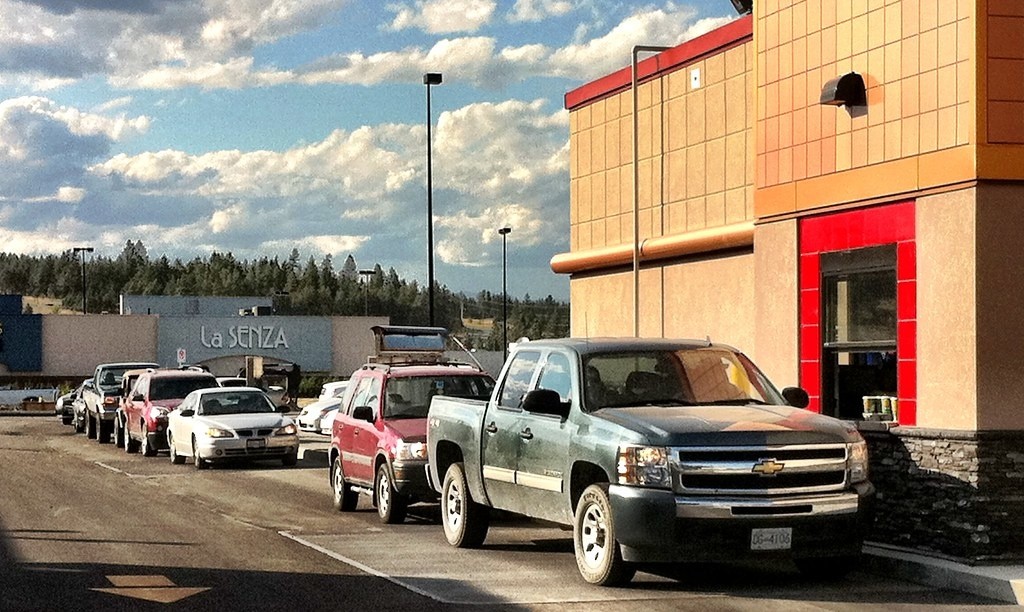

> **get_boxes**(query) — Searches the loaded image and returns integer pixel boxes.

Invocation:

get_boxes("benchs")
[21,401,55,411]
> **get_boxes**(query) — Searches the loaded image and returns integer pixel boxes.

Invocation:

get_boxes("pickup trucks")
[425,336,872,589]
[83,362,161,444]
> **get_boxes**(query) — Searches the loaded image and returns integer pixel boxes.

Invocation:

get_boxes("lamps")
[820,71,869,107]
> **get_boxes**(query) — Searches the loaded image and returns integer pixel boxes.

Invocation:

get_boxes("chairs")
[389,393,408,416]
[204,399,222,411]
[238,399,253,412]
[628,371,664,400]
[105,372,116,382]
[426,389,465,411]
[583,366,606,407]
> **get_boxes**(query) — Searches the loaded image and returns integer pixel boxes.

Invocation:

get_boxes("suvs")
[122,366,222,459]
[328,351,498,527]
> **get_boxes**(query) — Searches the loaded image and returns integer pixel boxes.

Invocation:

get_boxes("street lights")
[73,247,94,314]
[498,226,512,361]
[360,270,377,317]
[423,72,443,324]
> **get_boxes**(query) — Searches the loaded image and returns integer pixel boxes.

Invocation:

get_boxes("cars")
[216,376,248,388]
[263,381,350,436]
[54,377,94,434]
[166,387,300,470]
